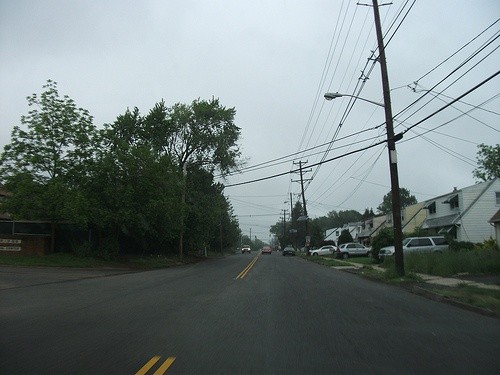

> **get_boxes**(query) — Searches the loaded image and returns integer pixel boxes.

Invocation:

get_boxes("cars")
[241,244,251,254]
[261,245,273,255]
[282,247,296,256]
[309,245,338,257]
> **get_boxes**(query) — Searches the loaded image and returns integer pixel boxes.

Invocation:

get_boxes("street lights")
[178,159,223,261]
[323,91,404,277]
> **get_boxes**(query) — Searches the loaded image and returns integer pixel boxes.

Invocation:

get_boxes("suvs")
[336,243,373,259]
[377,236,450,264]
